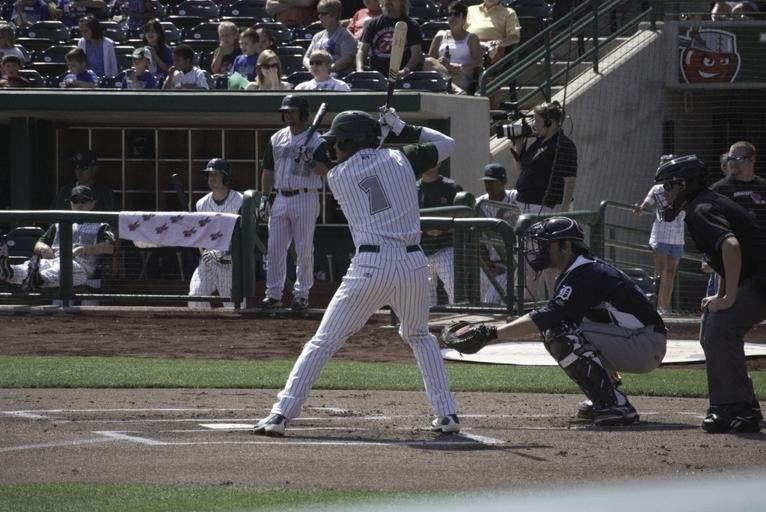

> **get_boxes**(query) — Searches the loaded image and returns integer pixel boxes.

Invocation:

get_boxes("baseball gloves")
[440,320,496,354]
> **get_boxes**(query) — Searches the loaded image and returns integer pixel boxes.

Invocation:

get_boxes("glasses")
[447,12,461,17]
[71,198,89,203]
[663,182,679,191]
[310,60,326,65]
[317,12,329,16]
[261,63,278,69]
[724,155,750,163]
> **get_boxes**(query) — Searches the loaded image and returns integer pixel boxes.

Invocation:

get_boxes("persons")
[257,95,331,307]
[414,102,577,305]
[447,216,668,427]
[711,0,761,21]
[630,139,766,433]
[186,158,246,308]
[0,0,518,95]
[49,152,115,308]
[252,111,461,442]
[0,185,115,289]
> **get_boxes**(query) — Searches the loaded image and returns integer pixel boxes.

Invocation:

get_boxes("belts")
[277,188,307,196]
[221,259,231,264]
[360,245,421,252]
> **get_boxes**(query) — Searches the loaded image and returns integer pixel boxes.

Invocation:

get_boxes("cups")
[111,76,121,87]
[99,76,111,87]
[216,77,228,89]
[46,78,57,87]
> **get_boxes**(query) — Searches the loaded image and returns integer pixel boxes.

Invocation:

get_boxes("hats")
[126,48,152,64]
[64,185,94,202]
[480,164,506,181]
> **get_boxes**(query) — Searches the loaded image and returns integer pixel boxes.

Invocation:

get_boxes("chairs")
[0,226,46,297]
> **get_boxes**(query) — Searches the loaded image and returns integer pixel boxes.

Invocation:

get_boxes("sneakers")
[262,298,283,307]
[702,408,759,433]
[21,254,40,293]
[595,405,638,424]
[291,296,310,310]
[578,401,605,419]
[0,246,13,283]
[253,413,287,436]
[431,414,460,432]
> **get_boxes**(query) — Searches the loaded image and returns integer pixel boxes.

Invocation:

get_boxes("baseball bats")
[296,103,329,162]
[386,21,408,110]
[171,172,204,257]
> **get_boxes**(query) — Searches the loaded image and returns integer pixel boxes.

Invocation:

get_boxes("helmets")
[525,216,584,281]
[320,110,381,150]
[201,158,232,185]
[279,95,308,123]
[653,154,707,222]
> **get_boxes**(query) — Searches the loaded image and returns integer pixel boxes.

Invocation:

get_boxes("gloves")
[259,196,271,222]
[296,144,314,165]
[379,105,398,128]
[202,249,223,265]
